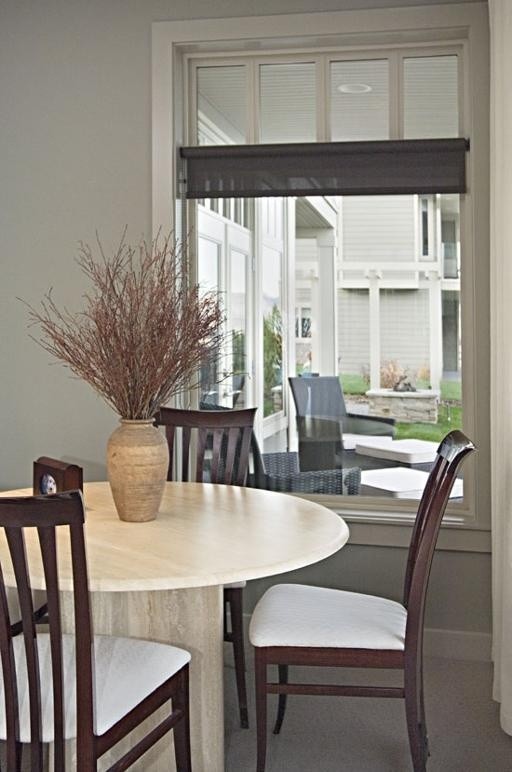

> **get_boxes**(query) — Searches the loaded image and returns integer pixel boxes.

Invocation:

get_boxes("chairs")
[202,395,362,495]
[156,402,261,731]
[245,425,481,772]
[0,482,190,769]
[285,374,398,470]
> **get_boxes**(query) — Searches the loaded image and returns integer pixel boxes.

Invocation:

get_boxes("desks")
[351,436,445,472]
[0,464,350,771]
[347,462,465,501]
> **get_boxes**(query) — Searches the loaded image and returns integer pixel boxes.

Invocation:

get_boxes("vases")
[104,415,172,525]
